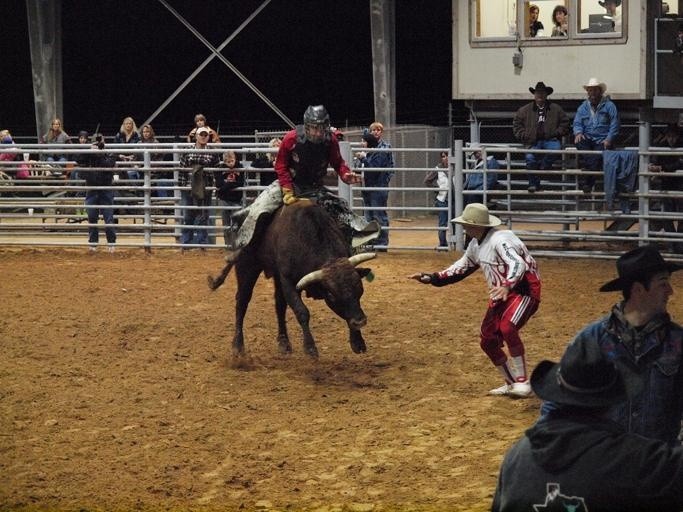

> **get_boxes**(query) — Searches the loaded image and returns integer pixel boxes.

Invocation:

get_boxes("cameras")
[94,136,104,149]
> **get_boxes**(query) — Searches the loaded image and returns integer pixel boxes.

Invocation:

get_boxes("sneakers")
[488,384,511,395]
[528,186,537,193]
[224,248,249,263]
[582,185,593,193]
[508,380,535,397]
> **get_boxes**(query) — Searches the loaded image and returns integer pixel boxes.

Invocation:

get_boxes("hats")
[450,203,502,227]
[467,142,486,157]
[599,245,683,292]
[529,82,553,95]
[91,134,105,144]
[361,134,379,147]
[662,122,682,134]
[530,333,628,407]
[196,127,210,135]
[583,78,607,94]
[599,0,621,7]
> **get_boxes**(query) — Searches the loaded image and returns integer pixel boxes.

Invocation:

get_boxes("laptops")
[589,13,614,34]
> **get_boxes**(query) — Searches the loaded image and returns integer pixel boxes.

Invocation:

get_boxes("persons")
[353,132,389,253]
[370,122,396,181]
[600,1,622,31]
[463,143,499,203]
[129,126,171,215]
[250,138,282,194]
[553,6,567,37]
[0,131,19,160]
[406,203,541,398]
[178,128,220,250]
[529,4,544,37]
[217,151,246,249]
[43,119,71,167]
[113,117,139,160]
[83,135,116,252]
[78,131,88,144]
[649,123,683,255]
[491,338,683,512]
[541,247,682,443]
[514,82,569,191]
[424,151,455,247]
[186,114,220,143]
[573,78,619,198]
[224,106,381,263]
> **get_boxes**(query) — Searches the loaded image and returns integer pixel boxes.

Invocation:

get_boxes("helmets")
[303,105,330,144]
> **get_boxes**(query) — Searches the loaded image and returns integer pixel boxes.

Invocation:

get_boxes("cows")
[205,194,379,365]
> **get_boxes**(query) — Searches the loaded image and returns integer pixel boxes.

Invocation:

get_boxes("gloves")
[282,187,298,205]
